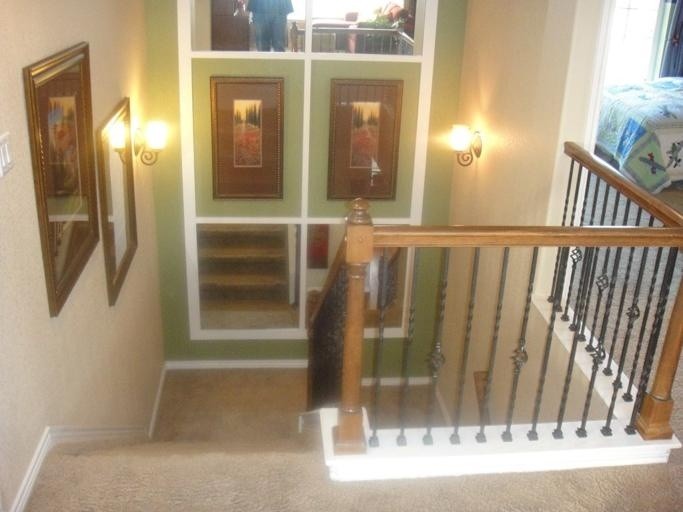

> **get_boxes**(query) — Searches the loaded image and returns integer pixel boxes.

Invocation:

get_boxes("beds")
[595,75,683,196]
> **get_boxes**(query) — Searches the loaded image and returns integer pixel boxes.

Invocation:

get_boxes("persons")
[245,0,294,51]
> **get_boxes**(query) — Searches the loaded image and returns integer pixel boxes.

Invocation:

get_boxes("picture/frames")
[96,97,139,306]
[23,41,103,318]
[329,79,404,201]
[209,76,285,200]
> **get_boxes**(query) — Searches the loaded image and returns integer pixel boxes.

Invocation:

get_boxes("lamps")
[134,123,170,166]
[450,122,482,167]
